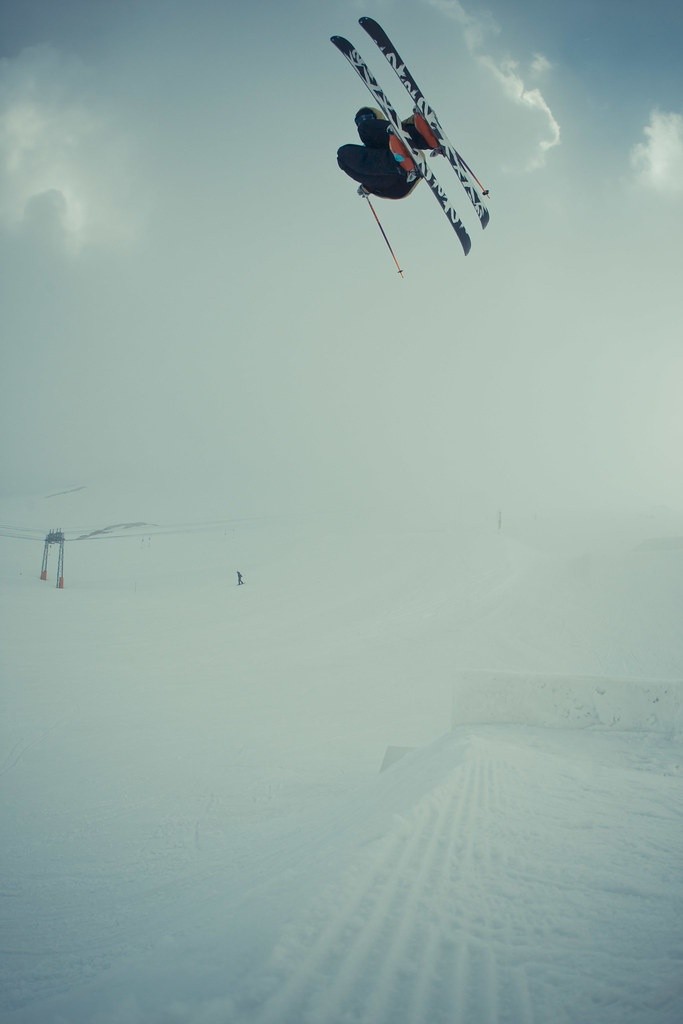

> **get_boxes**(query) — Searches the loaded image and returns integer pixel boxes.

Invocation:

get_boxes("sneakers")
[413,107,441,158]
[387,126,417,182]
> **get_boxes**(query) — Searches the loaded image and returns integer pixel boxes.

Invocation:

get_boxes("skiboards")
[330,17,489,256]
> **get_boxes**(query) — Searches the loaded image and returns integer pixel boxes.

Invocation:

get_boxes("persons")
[337,106,440,199]
[236,571,243,586]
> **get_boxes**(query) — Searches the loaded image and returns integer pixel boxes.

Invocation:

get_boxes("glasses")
[354,114,373,124]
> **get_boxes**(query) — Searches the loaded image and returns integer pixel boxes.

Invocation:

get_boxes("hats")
[356,107,385,120]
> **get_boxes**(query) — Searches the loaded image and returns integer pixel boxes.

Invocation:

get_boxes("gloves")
[357,185,370,198]
